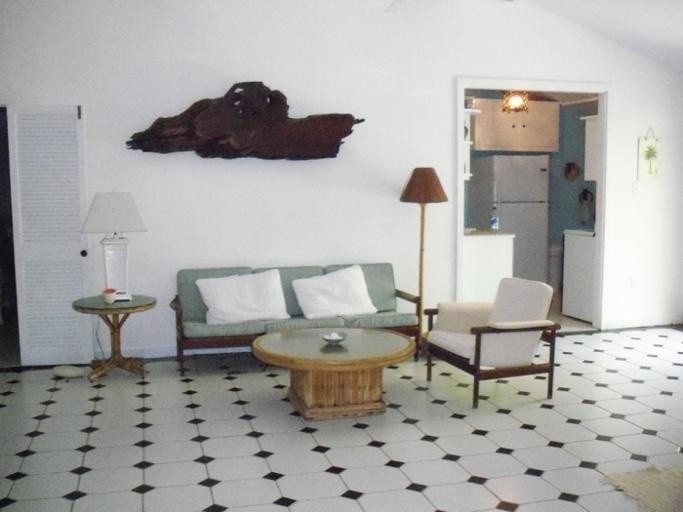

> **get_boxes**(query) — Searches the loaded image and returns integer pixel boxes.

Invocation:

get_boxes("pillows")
[195,263,379,324]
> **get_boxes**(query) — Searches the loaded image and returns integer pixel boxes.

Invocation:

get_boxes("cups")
[103,288,116,304]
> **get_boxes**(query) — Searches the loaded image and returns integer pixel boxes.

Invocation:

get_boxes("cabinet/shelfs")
[466,97,559,153]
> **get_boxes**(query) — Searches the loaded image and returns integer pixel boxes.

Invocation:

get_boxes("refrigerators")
[467,154,550,284]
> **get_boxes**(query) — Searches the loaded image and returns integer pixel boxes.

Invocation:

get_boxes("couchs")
[168,262,422,376]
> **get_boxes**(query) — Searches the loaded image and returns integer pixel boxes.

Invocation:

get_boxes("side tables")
[72,295,157,382]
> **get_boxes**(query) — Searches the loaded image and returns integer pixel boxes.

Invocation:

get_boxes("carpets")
[605,466,683,512]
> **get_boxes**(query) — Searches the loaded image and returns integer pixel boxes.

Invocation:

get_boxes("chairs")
[423,277,561,409]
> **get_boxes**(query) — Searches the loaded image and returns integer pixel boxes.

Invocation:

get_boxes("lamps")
[399,168,450,361]
[81,190,148,301]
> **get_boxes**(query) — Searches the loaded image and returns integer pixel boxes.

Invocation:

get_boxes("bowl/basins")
[319,330,348,345]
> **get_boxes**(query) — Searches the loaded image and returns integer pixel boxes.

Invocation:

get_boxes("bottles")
[488,206,500,234]
